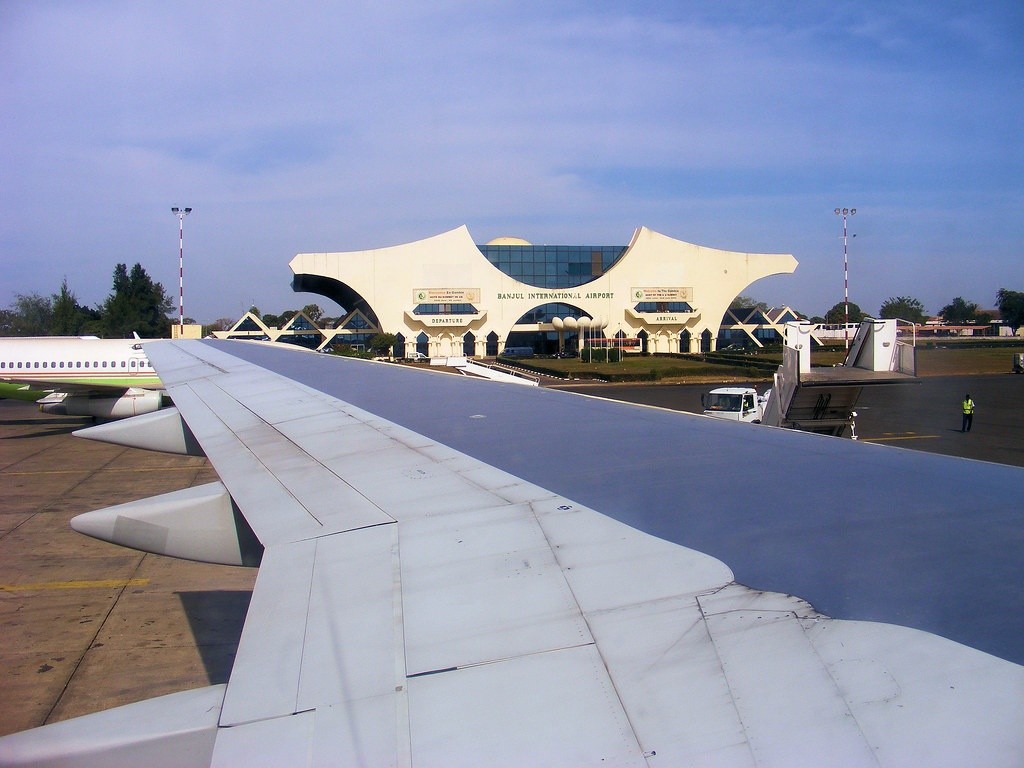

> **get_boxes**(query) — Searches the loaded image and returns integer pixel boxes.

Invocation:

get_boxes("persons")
[961,394,974,432]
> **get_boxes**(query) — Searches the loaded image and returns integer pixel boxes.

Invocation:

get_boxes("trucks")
[701,386,772,425]
[1012,353,1024,373]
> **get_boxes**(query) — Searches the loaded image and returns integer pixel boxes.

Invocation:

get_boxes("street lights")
[170,206,192,340]
[833,208,858,361]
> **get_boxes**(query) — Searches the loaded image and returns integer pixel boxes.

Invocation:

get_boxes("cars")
[721,342,751,350]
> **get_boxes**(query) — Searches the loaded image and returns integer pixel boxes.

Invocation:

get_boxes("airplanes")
[1,329,319,418]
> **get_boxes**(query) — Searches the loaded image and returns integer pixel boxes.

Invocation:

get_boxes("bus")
[574,337,642,355]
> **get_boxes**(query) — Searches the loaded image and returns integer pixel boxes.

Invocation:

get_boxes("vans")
[498,346,534,357]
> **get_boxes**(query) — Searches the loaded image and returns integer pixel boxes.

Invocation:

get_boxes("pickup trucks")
[405,353,431,361]
